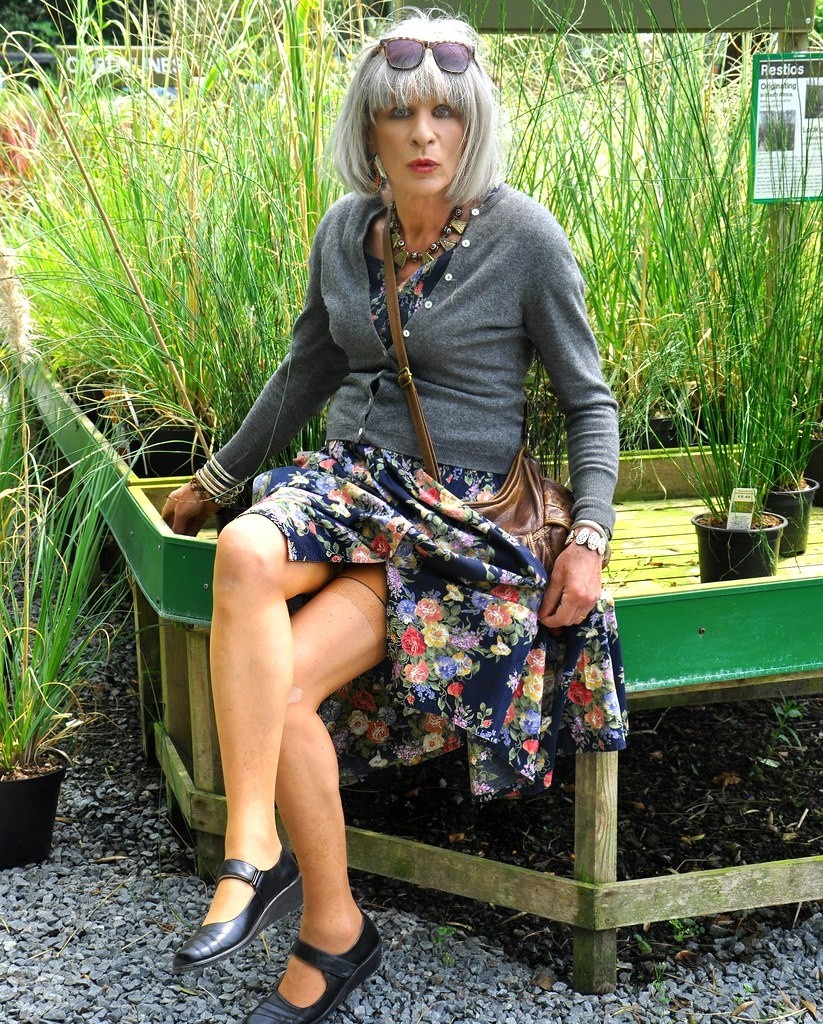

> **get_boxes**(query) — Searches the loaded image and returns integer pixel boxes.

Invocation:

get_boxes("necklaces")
[389,199,466,269]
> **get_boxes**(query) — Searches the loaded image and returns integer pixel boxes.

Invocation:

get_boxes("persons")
[162,11,630,1024]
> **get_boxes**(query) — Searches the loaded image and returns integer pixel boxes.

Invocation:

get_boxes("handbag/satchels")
[464,451,612,579]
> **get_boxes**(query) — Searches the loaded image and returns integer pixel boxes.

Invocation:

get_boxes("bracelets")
[189,455,246,507]
[563,527,612,571]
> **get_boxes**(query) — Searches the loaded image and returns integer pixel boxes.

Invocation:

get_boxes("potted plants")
[0,388,151,872]
[591,0,823,584]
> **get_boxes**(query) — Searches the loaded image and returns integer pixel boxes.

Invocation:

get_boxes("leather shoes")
[243,909,382,1024]
[172,846,308,970]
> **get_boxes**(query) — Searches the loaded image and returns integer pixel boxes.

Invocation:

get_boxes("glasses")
[371,37,475,74]
